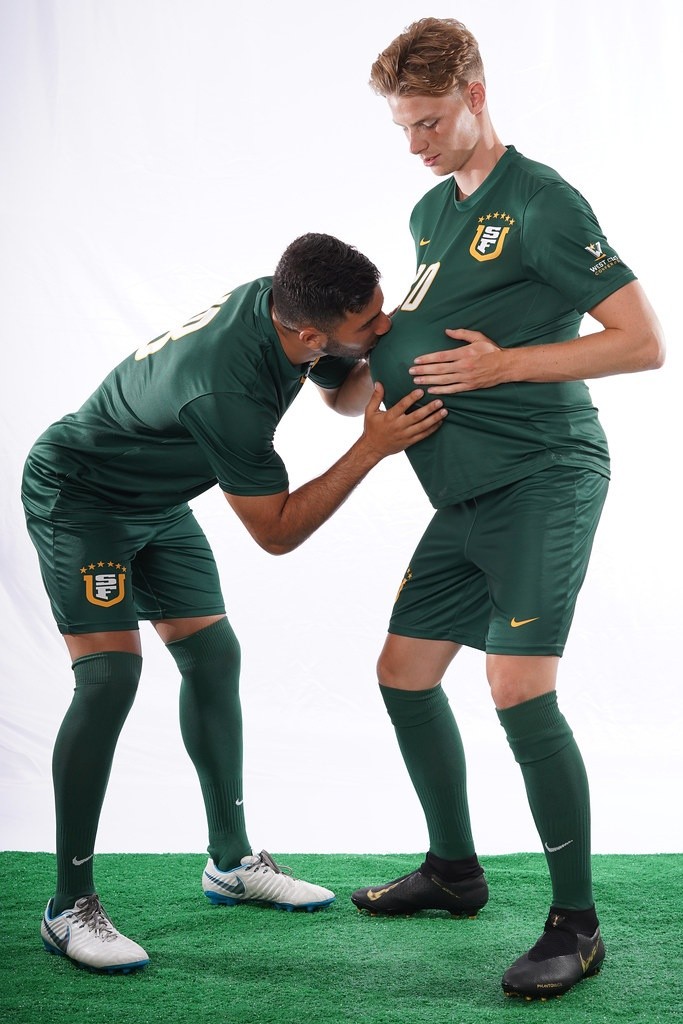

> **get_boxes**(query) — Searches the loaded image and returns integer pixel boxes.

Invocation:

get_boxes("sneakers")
[500,913,604,1001]
[202,849,335,913]
[350,854,489,919]
[41,892,149,974]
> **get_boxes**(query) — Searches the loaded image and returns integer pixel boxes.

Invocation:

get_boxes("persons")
[21,234,446,975]
[370,19,663,1003]
